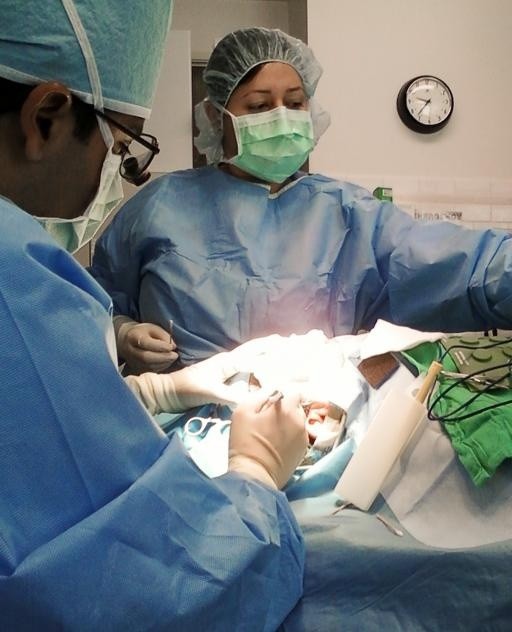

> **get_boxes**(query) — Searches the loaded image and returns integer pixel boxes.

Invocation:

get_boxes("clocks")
[397,74,453,134]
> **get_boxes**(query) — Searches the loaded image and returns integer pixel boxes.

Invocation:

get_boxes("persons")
[0,1,312,630]
[83,23,511,380]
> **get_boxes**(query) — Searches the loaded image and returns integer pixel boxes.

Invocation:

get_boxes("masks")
[212,102,313,183]
[30,0,124,254]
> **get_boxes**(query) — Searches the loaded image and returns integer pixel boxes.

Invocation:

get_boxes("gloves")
[149,353,263,412]
[117,317,179,374]
[229,387,308,490]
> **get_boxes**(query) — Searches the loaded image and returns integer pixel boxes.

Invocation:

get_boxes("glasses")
[73,93,159,186]
